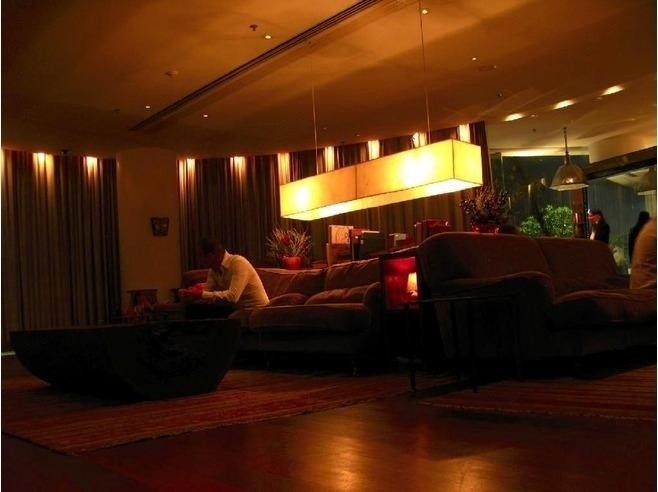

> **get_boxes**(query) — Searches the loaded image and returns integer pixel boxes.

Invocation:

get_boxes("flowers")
[264,223,314,267]
[457,182,512,223]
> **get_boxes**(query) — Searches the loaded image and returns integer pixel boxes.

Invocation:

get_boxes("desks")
[8,317,243,407]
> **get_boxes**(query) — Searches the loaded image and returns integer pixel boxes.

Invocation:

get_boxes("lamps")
[150,218,168,236]
[548,126,589,193]
[278,0,483,222]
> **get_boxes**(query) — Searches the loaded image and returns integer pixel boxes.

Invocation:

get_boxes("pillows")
[180,268,210,289]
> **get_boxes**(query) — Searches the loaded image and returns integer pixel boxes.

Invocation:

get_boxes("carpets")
[2,367,460,456]
[415,362,656,425]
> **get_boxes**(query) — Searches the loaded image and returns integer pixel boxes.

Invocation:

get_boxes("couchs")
[407,233,656,393]
[233,257,381,377]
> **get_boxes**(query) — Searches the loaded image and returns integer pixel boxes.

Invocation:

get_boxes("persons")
[178,239,270,337]
[628,212,649,263]
[588,209,609,244]
[629,217,657,290]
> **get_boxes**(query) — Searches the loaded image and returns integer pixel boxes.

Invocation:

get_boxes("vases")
[471,218,503,233]
[278,256,302,270]
